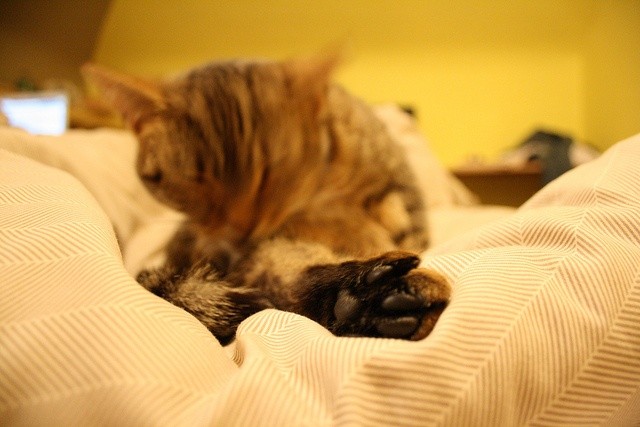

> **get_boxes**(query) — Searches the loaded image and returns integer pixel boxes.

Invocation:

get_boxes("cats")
[69,53,453,341]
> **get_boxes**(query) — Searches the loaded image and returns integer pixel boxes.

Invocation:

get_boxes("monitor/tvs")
[0,87,72,138]
